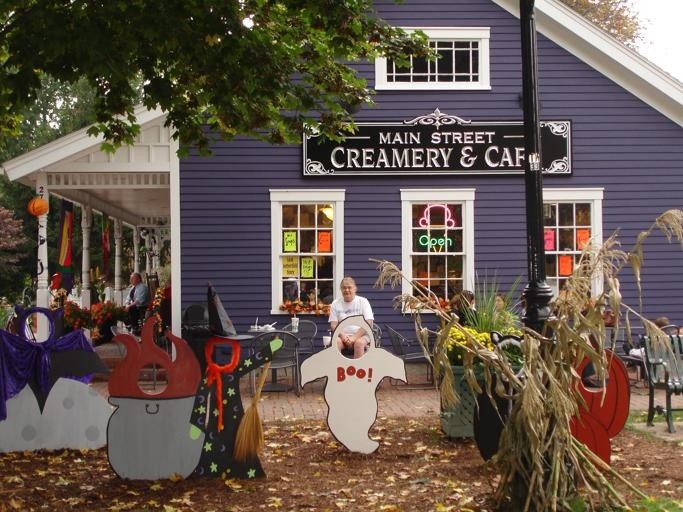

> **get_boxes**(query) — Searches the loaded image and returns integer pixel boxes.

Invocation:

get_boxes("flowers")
[62,286,169,345]
[436,323,523,367]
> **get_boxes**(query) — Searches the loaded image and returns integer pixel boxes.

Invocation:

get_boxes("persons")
[488,293,509,329]
[123,272,148,336]
[622,316,670,360]
[458,290,479,328]
[444,294,464,327]
[327,277,374,359]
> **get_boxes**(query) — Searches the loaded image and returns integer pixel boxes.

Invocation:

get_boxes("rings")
[347,340,349,342]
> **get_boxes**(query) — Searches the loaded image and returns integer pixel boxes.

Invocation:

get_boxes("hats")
[192,284,255,343]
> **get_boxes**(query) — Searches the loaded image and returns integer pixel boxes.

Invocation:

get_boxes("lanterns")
[26,197,48,216]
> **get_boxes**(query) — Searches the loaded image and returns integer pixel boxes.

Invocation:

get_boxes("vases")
[438,364,491,444]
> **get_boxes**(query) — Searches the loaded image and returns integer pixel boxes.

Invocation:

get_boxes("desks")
[222,334,255,395]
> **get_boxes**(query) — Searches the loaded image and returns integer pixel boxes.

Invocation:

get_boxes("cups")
[290,318,299,334]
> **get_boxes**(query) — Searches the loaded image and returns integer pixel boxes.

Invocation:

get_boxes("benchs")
[640,333,683,434]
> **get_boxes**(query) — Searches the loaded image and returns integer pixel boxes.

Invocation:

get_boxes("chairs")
[107,325,170,391]
[384,322,435,391]
[615,323,678,383]
[248,318,318,397]
